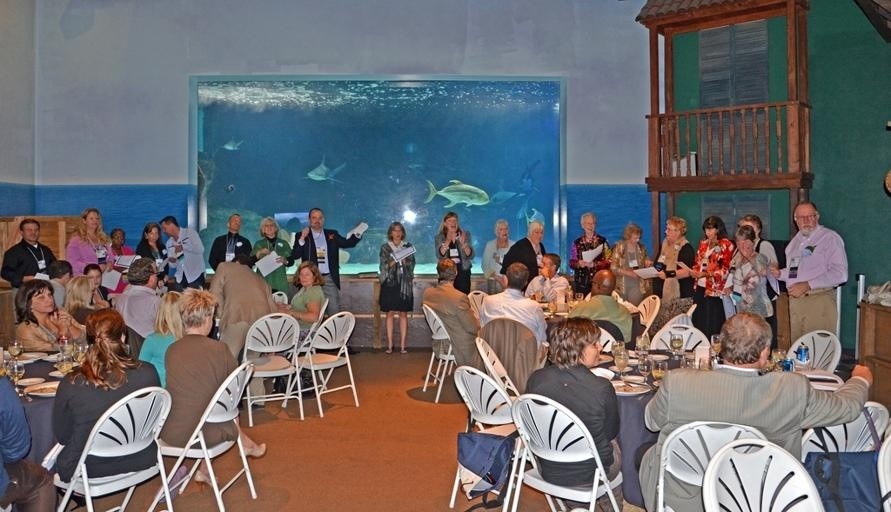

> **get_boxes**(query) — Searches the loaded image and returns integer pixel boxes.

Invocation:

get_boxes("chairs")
[701,437,827,512]
[448,366,526,511]
[464,289,728,351]
[422,302,455,403]
[271,292,333,390]
[510,390,624,511]
[473,315,544,400]
[786,325,891,512]
[239,311,305,428]
[285,311,360,418]
[145,358,258,511]
[54,385,175,511]
[655,420,769,511]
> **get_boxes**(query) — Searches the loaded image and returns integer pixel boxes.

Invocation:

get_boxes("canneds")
[782,358,794,372]
[796,346,809,362]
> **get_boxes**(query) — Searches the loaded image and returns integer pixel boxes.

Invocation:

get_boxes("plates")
[13,350,78,398]
[589,350,669,397]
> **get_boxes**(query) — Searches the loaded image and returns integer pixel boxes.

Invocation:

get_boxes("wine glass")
[610,334,786,386]
[0,337,26,390]
[56,335,94,375]
[534,287,584,315]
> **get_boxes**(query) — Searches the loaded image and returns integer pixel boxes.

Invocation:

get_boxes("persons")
[379,222,415,354]
[1,208,364,512]
[524,316,624,512]
[434,202,847,359]
[632,312,873,512]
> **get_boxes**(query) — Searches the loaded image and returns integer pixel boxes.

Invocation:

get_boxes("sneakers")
[347,346,361,355]
[251,403,265,412]
[400,345,408,355]
[385,345,395,354]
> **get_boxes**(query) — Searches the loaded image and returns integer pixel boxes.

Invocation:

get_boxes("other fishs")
[224,183,237,194]
[491,190,526,206]
[302,153,347,187]
[422,179,490,209]
[520,160,542,194]
[523,208,545,228]
[224,136,245,153]
[517,198,528,220]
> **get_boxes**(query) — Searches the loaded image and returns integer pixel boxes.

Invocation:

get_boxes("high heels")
[239,442,266,459]
[194,470,219,492]
